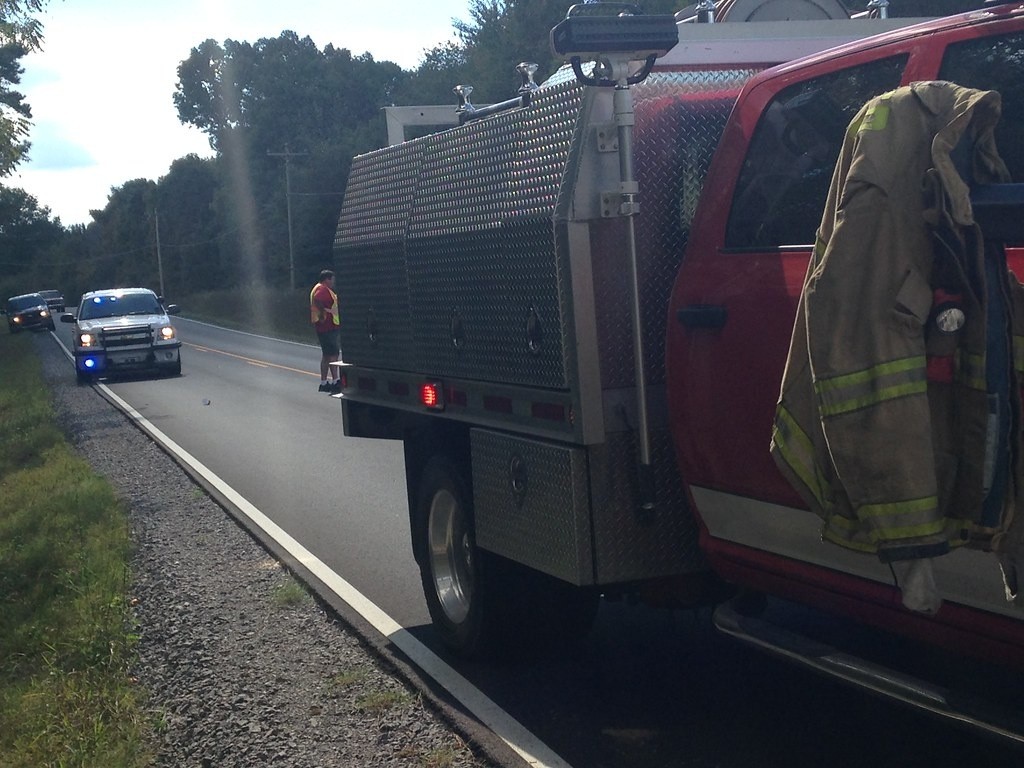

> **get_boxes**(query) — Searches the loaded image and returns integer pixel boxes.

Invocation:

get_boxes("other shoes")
[318,381,333,392]
[330,380,344,396]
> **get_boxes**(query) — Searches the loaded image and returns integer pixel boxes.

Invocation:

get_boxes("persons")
[309,270,341,393]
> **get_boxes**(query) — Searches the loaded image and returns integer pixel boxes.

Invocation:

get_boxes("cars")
[38,289,67,313]
[60,287,183,381]
[2,293,56,334]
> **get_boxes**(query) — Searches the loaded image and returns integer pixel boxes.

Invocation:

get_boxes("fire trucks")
[317,1,1024,768]
